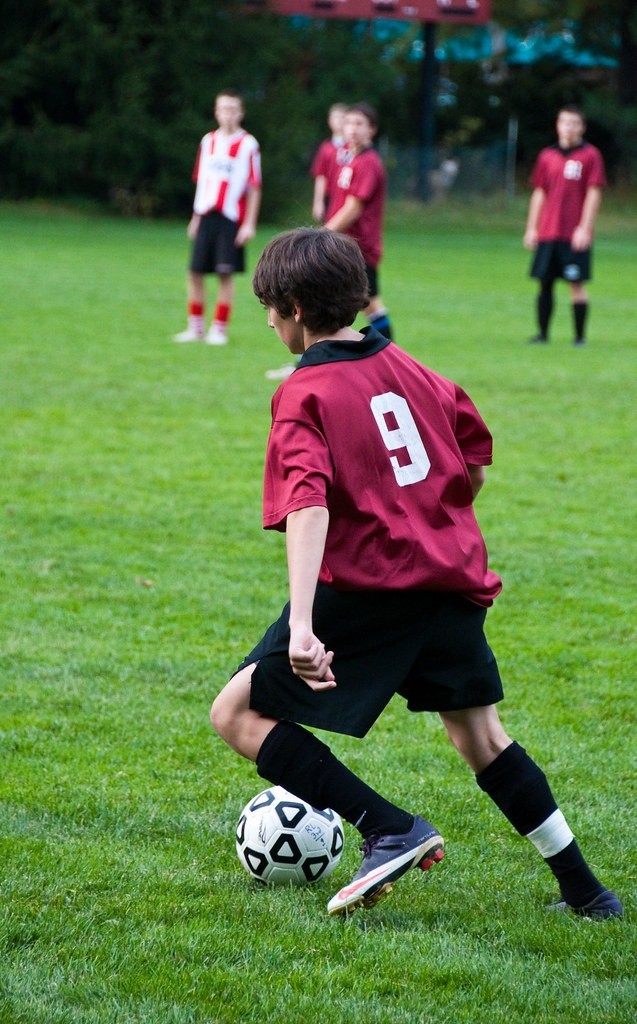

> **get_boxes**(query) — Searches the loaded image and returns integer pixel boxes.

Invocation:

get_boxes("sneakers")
[543,889,624,920]
[323,812,448,921]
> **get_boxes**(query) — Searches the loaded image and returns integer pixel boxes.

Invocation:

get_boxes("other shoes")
[265,364,299,383]
[206,328,229,347]
[171,326,201,344]
[573,338,588,347]
[530,332,550,343]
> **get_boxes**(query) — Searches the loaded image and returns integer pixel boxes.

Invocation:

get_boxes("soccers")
[234,783,345,886]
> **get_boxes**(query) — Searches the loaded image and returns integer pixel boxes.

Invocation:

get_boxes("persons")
[309,100,602,347]
[178,91,262,343]
[211,226,625,925]
